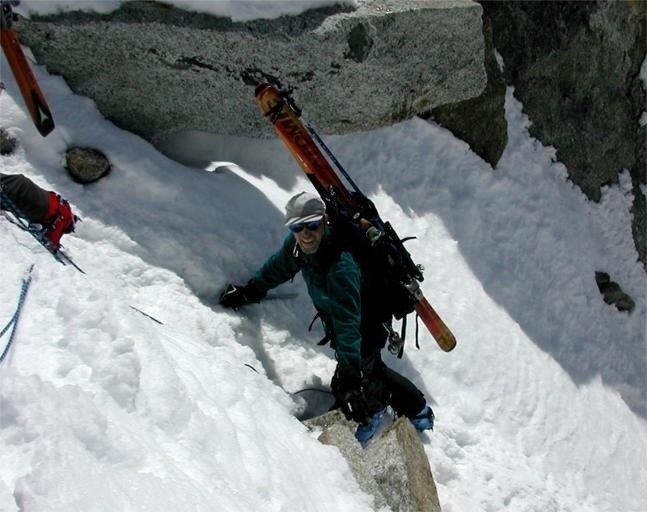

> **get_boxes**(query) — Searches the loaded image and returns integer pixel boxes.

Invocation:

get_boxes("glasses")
[289,222,320,234]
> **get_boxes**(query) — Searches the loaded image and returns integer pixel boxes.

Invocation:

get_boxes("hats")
[284,193,327,227]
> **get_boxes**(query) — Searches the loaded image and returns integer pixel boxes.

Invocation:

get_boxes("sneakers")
[412,404,435,430]
[355,407,389,444]
[41,194,74,254]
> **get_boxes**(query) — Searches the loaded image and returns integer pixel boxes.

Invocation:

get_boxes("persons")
[219,192,433,448]
[0,174,78,254]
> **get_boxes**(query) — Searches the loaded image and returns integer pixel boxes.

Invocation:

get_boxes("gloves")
[336,380,372,426]
[220,278,264,310]
